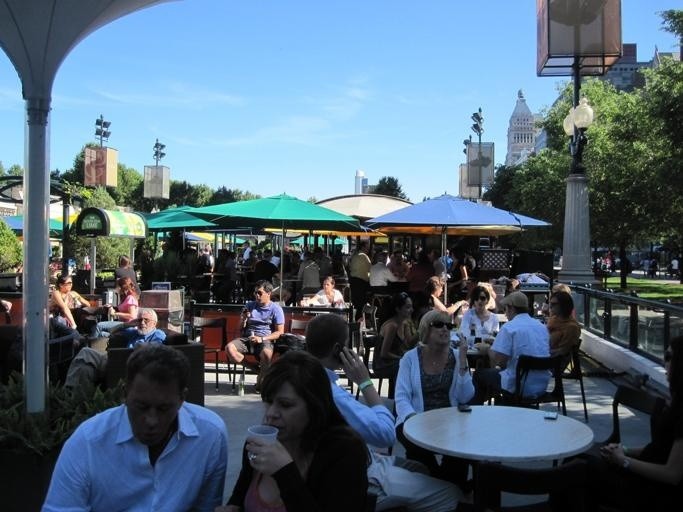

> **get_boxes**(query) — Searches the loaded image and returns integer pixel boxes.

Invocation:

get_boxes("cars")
[627,255,640,270]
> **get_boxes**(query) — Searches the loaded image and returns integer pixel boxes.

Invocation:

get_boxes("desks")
[402,405,594,512]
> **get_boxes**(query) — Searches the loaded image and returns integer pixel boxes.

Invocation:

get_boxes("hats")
[499,291,529,308]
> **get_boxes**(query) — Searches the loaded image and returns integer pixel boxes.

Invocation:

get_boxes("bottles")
[238,379,245,396]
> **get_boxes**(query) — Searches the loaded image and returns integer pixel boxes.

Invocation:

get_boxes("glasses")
[475,296,487,301]
[254,291,262,296]
[429,321,453,330]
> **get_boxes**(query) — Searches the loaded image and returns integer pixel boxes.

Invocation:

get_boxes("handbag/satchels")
[274,333,306,352]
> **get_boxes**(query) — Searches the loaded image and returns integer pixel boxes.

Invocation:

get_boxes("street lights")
[152,139,166,212]
[462,135,473,201]
[561,99,595,282]
[471,107,487,200]
[93,114,110,191]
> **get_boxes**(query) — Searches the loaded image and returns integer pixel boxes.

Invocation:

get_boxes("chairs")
[335,322,360,394]
[86,327,206,407]
[190,315,231,392]
[561,384,666,463]
[356,303,379,337]
[356,335,392,401]
[0,324,74,386]
[81,305,112,348]
[558,339,589,423]
[289,319,307,334]
[174,256,352,305]
[231,327,280,395]
[490,354,570,416]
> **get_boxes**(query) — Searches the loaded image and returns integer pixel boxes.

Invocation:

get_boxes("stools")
[370,294,394,313]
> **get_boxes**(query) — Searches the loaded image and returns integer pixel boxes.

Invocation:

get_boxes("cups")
[245,425,280,441]
[466,335,474,349]
[301,296,349,310]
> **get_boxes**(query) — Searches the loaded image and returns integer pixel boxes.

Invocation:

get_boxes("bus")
[591,249,620,272]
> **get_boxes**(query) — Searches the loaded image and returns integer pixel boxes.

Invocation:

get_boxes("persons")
[1,239,683,512]
